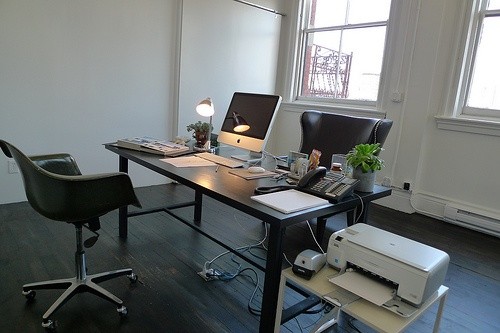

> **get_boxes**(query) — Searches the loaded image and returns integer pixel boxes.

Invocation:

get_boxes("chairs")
[298,111,393,246]
[0,138,142,330]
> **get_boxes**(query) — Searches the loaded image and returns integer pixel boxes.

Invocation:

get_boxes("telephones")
[297,166,360,203]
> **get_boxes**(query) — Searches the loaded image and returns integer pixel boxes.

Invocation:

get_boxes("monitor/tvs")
[217,92,282,162]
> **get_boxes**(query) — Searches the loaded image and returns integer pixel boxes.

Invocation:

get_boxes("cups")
[332,154,353,178]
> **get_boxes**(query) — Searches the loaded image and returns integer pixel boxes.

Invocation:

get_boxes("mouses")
[248,166,265,173]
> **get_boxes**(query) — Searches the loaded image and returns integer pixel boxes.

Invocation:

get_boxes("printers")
[326,223,449,316]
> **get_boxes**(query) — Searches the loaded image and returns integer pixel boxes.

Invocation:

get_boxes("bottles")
[330,163,342,175]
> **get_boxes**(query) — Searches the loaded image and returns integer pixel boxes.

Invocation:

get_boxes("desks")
[103,140,392,333]
[274,263,449,333]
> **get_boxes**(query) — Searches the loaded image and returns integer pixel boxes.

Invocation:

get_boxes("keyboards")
[193,152,244,169]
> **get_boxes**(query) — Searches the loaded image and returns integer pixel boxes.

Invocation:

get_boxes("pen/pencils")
[216,165,220,172]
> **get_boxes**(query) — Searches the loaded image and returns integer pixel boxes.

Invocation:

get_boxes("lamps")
[193,97,215,152]
[226,111,251,132]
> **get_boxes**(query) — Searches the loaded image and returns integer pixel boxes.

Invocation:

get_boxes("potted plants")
[186,120,214,145]
[345,143,385,194]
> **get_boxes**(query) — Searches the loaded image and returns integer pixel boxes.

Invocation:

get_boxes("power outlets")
[8,159,18,174]
[197,269,220,281]
